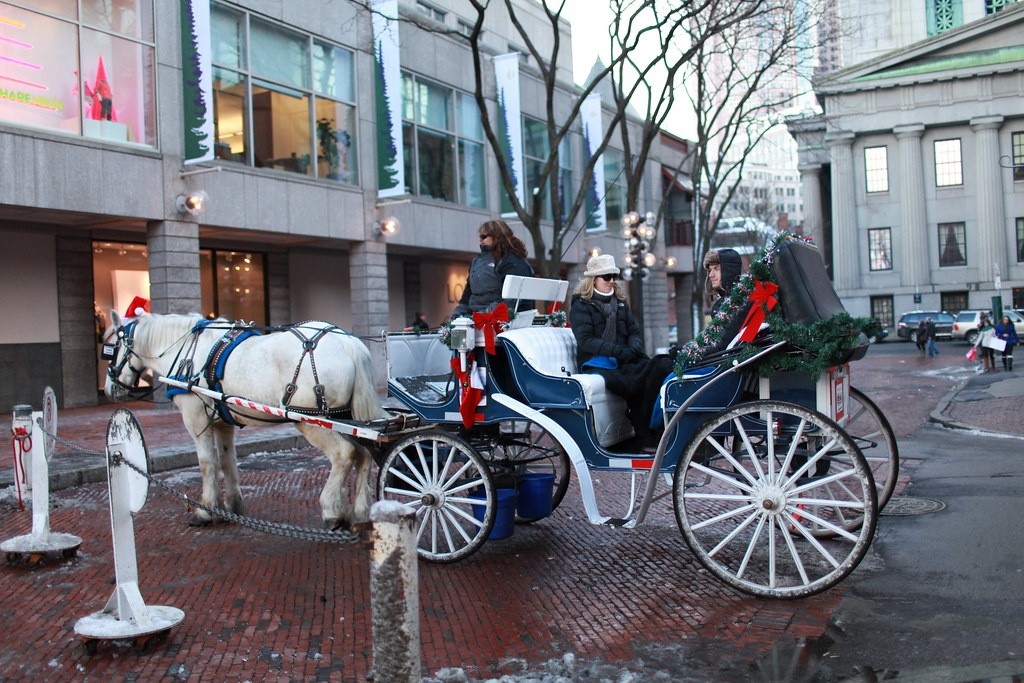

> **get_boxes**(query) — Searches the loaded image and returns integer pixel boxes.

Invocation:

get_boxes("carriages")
[101,235,899,603]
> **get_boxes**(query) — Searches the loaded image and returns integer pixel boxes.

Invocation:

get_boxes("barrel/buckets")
[510,443,557,519]
[467,465,517,541]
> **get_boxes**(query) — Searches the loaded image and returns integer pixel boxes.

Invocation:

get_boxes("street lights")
[622,210,656,354]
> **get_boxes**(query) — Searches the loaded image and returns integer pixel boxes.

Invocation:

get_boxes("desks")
[268,158,308,174]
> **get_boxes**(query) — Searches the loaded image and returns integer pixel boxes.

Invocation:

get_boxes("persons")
[569,253,658,452]
[916,317,940,360]
[995,315,1019,372]
[629,249,742,422]
[413,311,429,334]
[974,311,997,371]
[451,220,536,327]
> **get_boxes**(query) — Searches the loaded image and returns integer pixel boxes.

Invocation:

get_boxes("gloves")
[669,346,684,360]
[618,347,636,363]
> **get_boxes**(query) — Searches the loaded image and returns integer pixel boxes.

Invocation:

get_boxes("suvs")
[951,309,1024,346]
[897,311,957,343]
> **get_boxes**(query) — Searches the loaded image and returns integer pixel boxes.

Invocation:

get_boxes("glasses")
[479,234,491,240]
[596,275,617,282]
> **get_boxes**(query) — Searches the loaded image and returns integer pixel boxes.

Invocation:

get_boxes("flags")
[73,56,117,121]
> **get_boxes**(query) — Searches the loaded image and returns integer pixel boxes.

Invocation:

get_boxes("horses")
[100,308,383,545]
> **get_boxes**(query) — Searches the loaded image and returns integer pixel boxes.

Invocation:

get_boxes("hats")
[584,253,620,277]
[704,248,742,296]
[416,310,424,317]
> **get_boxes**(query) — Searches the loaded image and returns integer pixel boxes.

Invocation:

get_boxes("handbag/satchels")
[920,332,928,344]
[966,348,977,362]
[988,334,1007,351]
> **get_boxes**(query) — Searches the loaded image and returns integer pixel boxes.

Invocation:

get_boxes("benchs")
[458,274,569,347]
[650,321,772,433]
[496,327,636,448]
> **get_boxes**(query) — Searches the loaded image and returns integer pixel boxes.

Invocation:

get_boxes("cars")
[869,327,888,344]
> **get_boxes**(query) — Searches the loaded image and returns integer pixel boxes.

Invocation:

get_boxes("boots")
[1001,356,1007,371]
[1007,355,1013,371]
[990,357,996,370]
[984,358,989,372]
[626,408,659,453]
